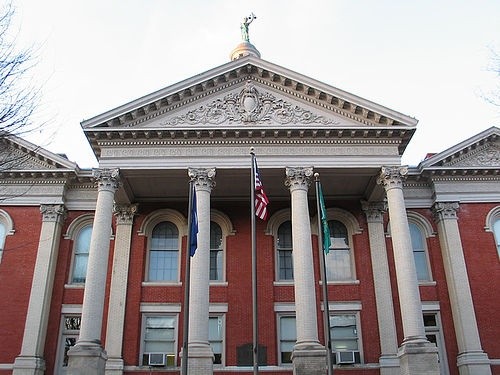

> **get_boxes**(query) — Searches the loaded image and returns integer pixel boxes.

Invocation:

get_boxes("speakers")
[149,353,165,365]
[335,352,355,365]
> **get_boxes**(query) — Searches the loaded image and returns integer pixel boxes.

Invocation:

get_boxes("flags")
[190,185,199,257]
[319,182,331,257]
[255,158,269,221]
